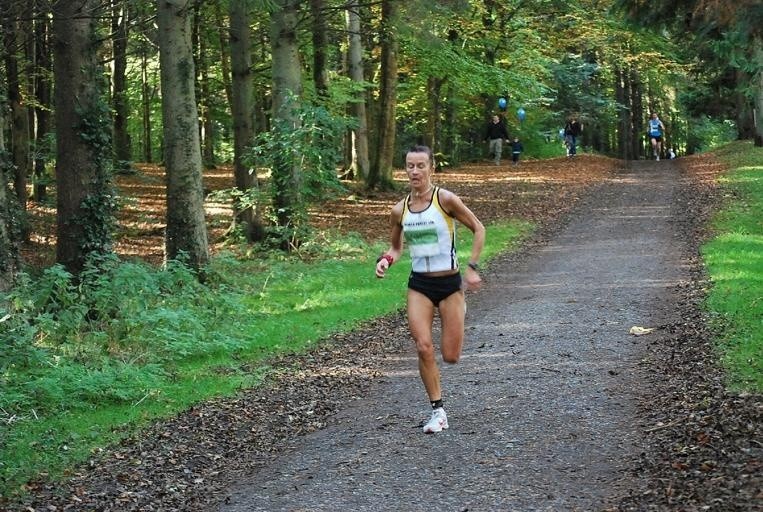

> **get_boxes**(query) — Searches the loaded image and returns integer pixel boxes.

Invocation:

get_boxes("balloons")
[498,98,507,109]
[517,108,525,120]
[559,128,565,138]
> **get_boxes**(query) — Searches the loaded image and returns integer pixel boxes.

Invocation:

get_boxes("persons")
[507,135,524,166]
[564,114,584,157]
[647,112,666,162]
[375,144,486,433]
[483,114,510,166]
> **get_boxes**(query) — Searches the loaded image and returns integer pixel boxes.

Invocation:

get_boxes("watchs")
[469,262,478,269]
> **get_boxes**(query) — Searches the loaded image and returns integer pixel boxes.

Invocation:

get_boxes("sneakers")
[423,408,449,433]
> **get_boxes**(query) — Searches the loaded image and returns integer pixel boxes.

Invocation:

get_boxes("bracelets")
[377,255,393,267]
